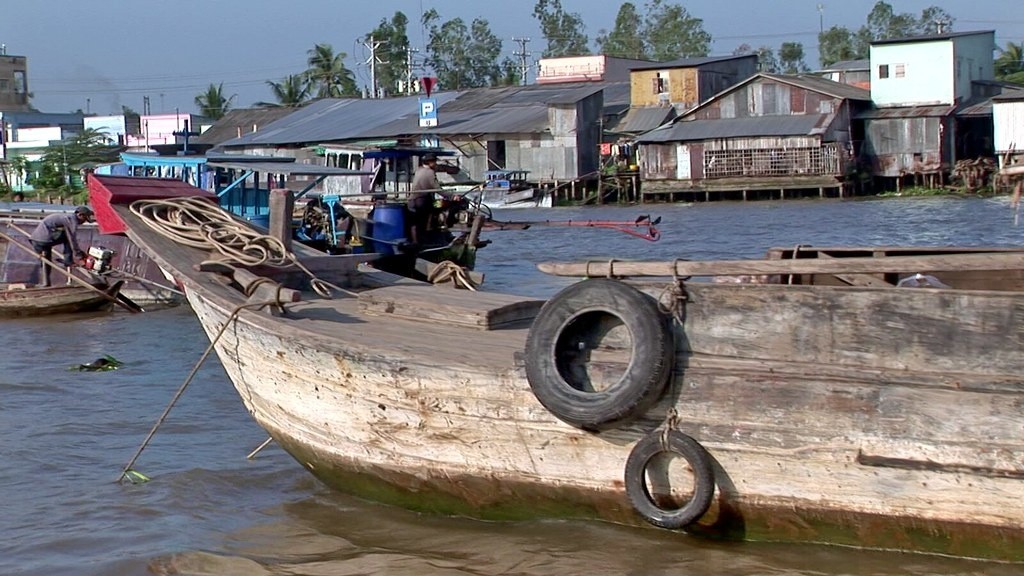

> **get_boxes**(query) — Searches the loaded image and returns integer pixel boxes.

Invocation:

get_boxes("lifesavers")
[623,428,715,529]
[522,277,669,434]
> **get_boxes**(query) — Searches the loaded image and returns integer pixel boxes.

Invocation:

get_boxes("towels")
[600,143,610,155]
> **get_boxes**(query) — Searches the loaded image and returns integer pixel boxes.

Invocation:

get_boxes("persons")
[406,153,461,246]
[12,193,23,201]
[29,207,93,286]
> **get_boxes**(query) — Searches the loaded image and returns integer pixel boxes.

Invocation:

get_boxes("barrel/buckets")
[244,214,269,231]
[371,202,406,253]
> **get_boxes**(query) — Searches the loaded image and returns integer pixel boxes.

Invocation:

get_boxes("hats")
[77,206,93,223]
[423,153,441,160]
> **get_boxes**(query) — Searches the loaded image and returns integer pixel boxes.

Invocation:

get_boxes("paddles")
[0,219,146,316]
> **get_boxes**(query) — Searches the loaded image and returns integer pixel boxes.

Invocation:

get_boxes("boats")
[94,140,663,277]
[0,278,126,318]
[1,214,186,302]
[436,168,554,209]
[82,169,1024,564]
